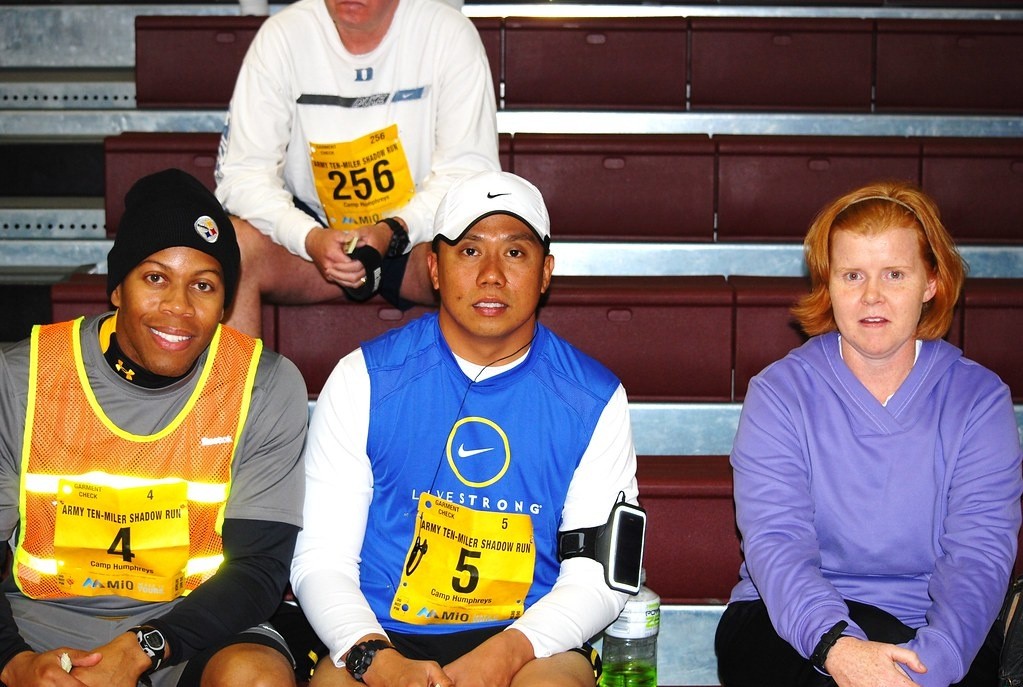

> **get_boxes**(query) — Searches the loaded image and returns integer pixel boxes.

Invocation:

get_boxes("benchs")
[49,8,1023,610]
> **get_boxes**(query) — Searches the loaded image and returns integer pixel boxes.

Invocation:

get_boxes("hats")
[431,170,552,255]
[107,168,241,312]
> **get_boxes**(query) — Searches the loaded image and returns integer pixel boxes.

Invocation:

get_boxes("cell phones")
[609,501,645,592]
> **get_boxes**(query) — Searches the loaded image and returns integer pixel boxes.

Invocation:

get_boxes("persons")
[211,0,499,353]
[715,180,1023,687]
[288,171,647,687]
[0,167,310,687]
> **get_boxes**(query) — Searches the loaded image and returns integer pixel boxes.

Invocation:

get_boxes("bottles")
[598,568,661,687]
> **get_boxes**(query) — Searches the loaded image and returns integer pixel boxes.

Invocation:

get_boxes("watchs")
[376,217,409,264]
[345,639,406,684]
[126,624,166,675]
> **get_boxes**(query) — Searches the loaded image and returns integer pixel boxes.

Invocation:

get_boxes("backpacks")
[998,573,1023,687]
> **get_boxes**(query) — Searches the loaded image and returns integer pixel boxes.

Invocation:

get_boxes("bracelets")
[811,620,852,674]
